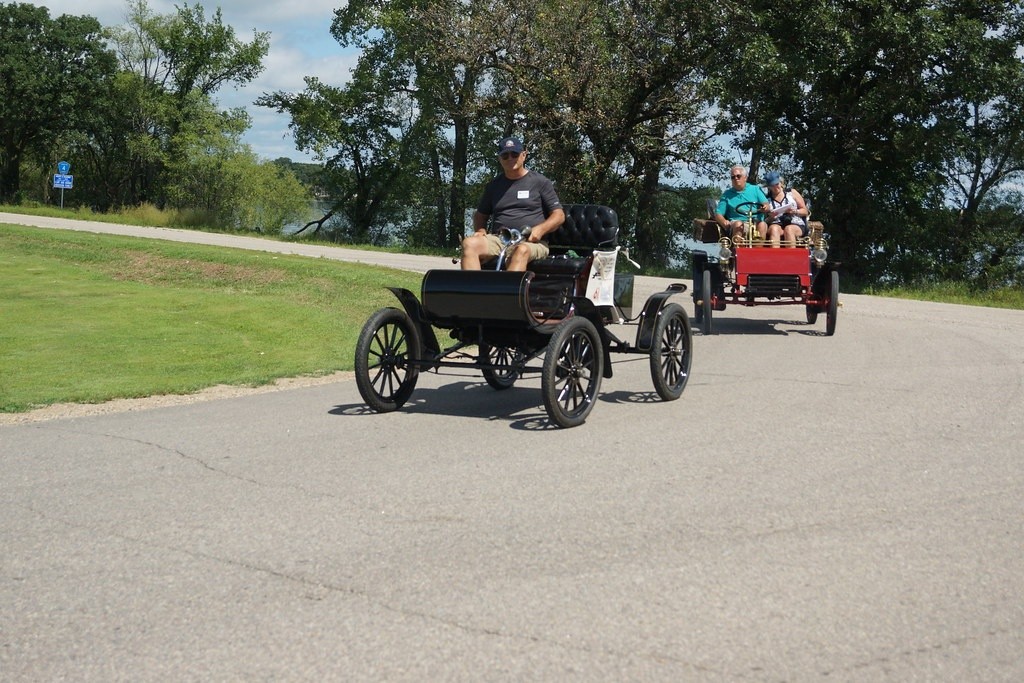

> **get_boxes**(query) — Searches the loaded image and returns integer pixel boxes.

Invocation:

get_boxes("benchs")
[708,199,813,248]
[484,204,618,317]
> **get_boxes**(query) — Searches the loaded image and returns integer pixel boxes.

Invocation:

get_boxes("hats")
[765,171,781,186]
[499,138,523,155]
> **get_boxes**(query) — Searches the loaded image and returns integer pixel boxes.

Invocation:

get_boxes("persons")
[715,164,770,248]
[461,138,565,271]
[763,172,810,248]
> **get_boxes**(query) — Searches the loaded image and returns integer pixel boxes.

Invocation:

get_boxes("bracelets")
[794,209,799,215]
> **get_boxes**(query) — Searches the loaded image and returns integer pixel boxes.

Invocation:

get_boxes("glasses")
[500,151,523,160]
[731,174,742,180]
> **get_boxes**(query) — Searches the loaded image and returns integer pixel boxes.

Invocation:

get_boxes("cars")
[354,204,695,428]
[689,197,843,339]
